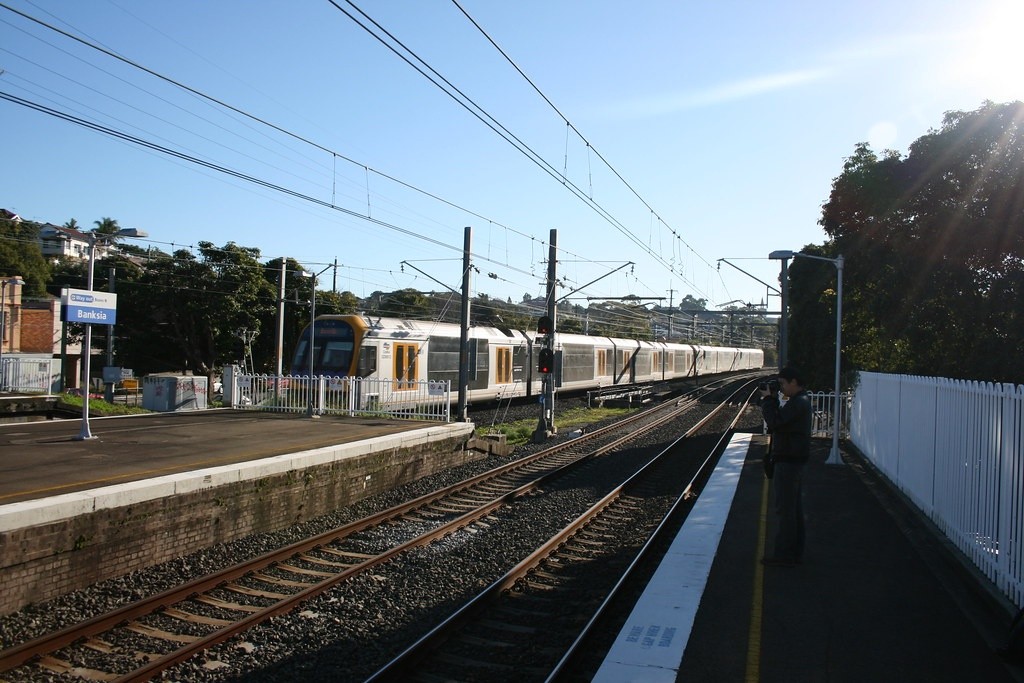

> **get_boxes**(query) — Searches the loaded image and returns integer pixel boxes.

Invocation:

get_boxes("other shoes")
[760,559,796,568]
[795,557,803,564]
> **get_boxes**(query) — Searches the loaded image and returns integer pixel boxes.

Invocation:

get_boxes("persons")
[758,369,813,567]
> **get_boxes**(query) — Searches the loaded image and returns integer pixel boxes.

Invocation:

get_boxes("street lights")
[768,250,845,466]
[0,278,26,374]
[72,227,150,440]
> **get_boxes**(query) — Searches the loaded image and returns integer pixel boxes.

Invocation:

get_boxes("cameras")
[759,380,776,391]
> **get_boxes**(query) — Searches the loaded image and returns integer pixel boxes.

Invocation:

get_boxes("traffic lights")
[538,316,553,335]
[537,348,552,373]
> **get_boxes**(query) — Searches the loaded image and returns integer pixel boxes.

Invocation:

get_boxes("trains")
[287,313,764,414]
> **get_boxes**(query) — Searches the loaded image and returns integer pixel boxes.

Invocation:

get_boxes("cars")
[213,379,224,394]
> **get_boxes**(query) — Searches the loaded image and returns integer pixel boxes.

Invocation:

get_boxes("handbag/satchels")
[763,454,775,479]
